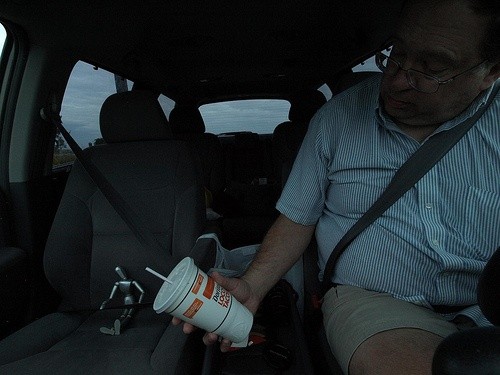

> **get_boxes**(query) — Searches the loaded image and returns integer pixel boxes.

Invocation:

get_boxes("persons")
[98,264,144,336]
[172,0,500,375]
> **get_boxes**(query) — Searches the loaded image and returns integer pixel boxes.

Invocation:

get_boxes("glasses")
[373,49,490,94]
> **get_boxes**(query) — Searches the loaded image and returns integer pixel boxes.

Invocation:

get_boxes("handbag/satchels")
[195,233,305,324]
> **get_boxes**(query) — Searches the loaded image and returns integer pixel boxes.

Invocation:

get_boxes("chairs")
[1,70,500,375]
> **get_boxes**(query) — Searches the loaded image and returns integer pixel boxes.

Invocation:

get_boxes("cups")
[153,257,254,343]
